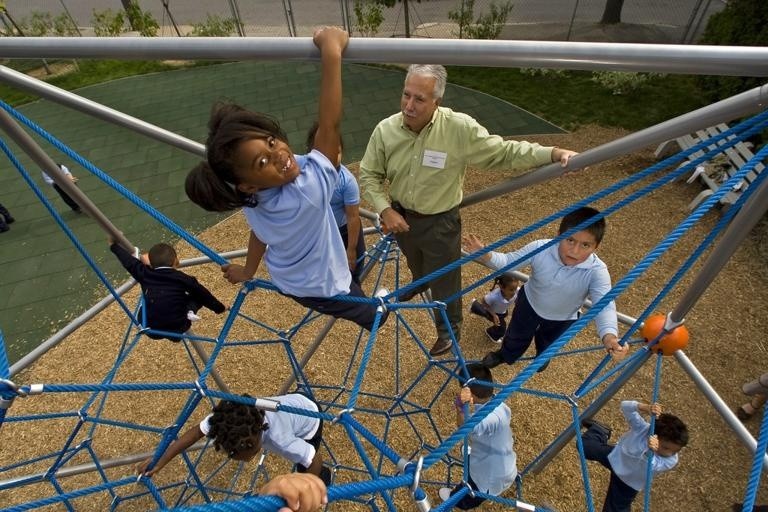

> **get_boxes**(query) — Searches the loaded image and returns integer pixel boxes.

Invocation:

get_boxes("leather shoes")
[429,334,459,356]
[737,401,760,421]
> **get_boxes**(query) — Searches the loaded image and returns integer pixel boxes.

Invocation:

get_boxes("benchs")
[653,120,766,212]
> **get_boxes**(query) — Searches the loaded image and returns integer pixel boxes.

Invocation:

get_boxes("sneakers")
[582,419,610,440]
[438,488,452,502]
[484,327,503,344]
[482,352,505,369]
[461,445,470,457]
[373,287,390,298]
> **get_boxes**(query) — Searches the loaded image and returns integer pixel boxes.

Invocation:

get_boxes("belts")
[405,210,427,219]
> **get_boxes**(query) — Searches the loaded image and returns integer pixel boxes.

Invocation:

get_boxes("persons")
[358,60,593,361]
[459,205,629,376]
[105,231,229,343]
[40,161,88,217]
[133,386,334,493]
[303,118,370,282]
[467,273,521,349]
[178,24,392,332]
[737,372,767,423]
[438,362,518,511]
[261,470,332,511]
[573,399,689,511]
[0,202,16,235]
